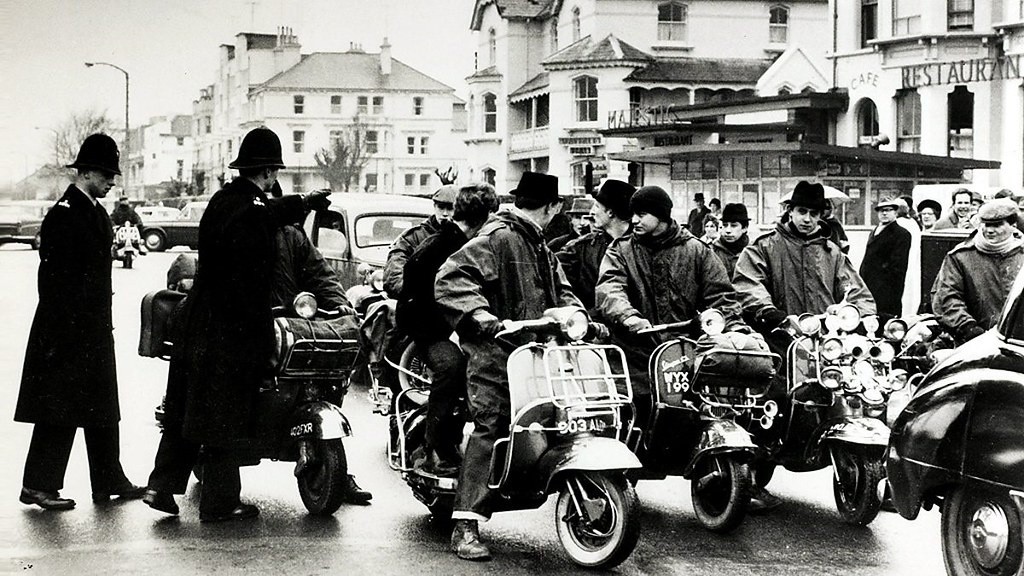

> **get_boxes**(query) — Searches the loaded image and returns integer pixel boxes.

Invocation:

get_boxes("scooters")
[153,292,364,519]
[769,289,959,532]
[110,221,145,269]
[614,304,785,535]
[385,307,645,573]
[349,268,434,410]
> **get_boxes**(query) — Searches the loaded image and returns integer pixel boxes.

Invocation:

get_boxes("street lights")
[84,61,129,197]
[34,126,59,169]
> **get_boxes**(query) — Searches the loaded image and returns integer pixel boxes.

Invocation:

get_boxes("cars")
[0,199,56,251]
[302,192,437,292]
[875,260,1023,576]
[134,200,208,252]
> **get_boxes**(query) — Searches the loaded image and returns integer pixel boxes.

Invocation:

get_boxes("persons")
[731,181,877,391]
[144,128,372,522]
[14,134,146,509]
[114,198,146,255]
[383,172,611,558]
[548,179,753,454]
[859,189,1024,343]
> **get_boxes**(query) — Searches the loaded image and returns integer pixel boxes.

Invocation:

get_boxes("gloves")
[964,326,984,342]
[762,309,786,329]
[302,188,332,210]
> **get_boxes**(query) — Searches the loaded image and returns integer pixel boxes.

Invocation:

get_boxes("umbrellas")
[778,185,852,210]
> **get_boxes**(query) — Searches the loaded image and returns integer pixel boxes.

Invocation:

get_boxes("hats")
[591,179,637,219]
[783,180,831,209]
[66,134,123,177]
[228,125,287,171]
[917,200,942,213]
[432,184,460,202]
[630,185,673,220]
[875,196,899,211]
[979,198,1018,223]
[995,189,1012,198]
[510,172,565,203]
[719,203,751,222]
[693,193,705,201]
[972,192,984,203]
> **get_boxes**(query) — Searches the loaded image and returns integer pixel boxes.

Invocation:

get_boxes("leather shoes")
[143,489,179,514]
[19,486,76,509]
[344,474,372,503]
[92,480,148,501]
[199,503,260,521]
[450,520,491,557]
